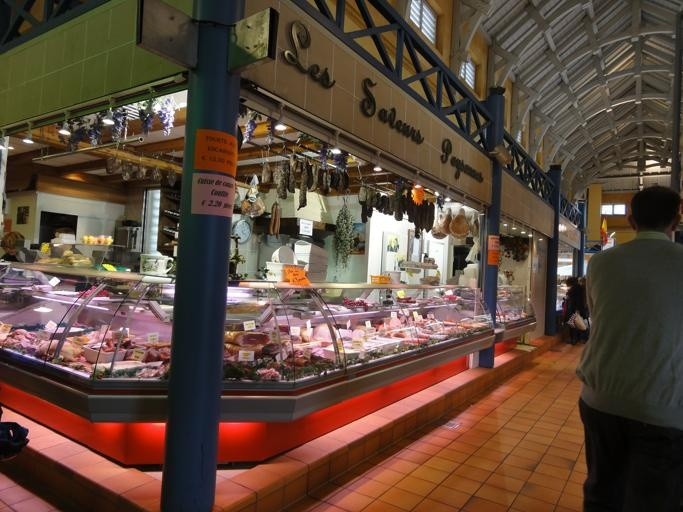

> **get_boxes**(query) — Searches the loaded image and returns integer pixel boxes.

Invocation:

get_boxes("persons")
[0,422,30,463]
[561,276,590,346]
[48,227,83,258]
[0,232,40,262]
[574,186,681,511]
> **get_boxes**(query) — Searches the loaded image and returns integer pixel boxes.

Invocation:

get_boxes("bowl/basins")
[271,246,297,265]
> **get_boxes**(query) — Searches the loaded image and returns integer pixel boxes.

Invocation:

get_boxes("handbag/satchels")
[574,313,587,331]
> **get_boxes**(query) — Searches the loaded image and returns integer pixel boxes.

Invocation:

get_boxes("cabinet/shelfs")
[1,262,538,466]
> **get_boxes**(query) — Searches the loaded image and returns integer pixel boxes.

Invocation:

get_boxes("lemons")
[81,235,114,245]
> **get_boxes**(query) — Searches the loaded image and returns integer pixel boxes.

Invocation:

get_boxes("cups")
[139,254,174,277]
